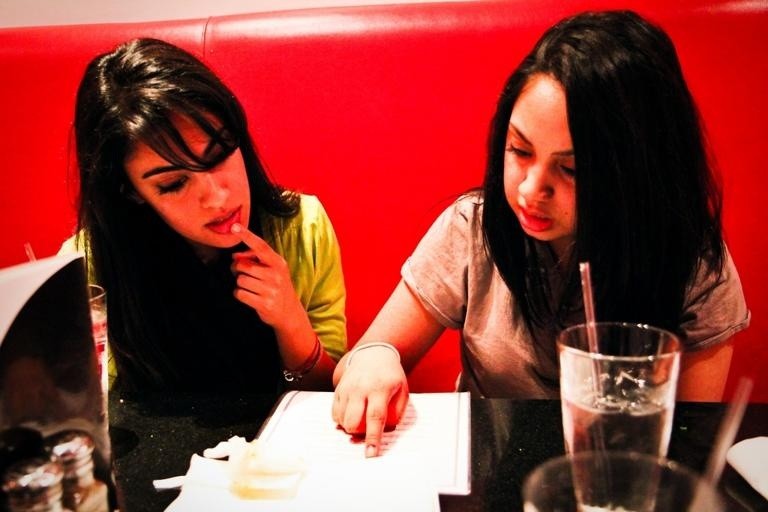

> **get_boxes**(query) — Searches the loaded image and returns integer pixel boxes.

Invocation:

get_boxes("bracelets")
[277,331,323,386]
[344,340,404,373]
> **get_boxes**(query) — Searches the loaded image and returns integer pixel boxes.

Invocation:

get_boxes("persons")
[50,37,348,399]
[329,7,753,457]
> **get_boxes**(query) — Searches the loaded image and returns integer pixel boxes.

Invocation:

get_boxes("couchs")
[0,1,768,405]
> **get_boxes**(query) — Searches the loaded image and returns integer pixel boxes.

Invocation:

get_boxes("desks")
[106,374,768,511]
